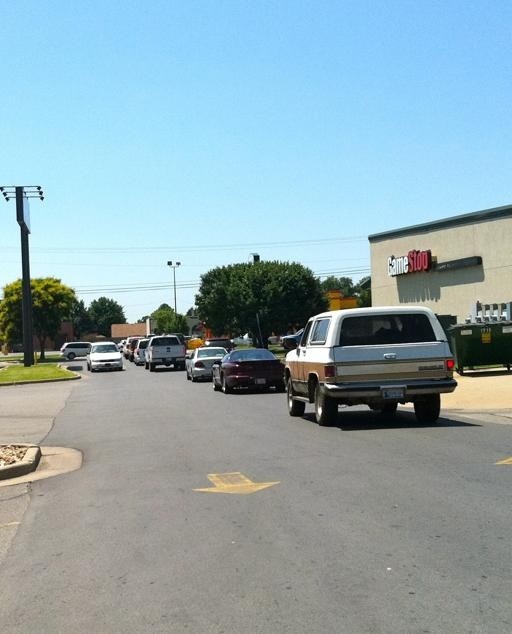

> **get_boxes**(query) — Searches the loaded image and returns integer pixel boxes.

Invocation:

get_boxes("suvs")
[283,305,457,424]
[60,341,92,360]
[116,332,192,372]
[280,327,305,349]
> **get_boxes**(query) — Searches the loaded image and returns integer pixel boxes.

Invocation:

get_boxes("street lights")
[0,185,45,366]
[167,260,182,332]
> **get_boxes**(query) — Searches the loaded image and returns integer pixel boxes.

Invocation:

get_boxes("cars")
[87,341,123,372]
[199,338,235,353]
[211,348,286,392]
[184,345,230,382]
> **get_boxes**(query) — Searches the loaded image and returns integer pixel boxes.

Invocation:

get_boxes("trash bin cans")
[446,321,512,374]
